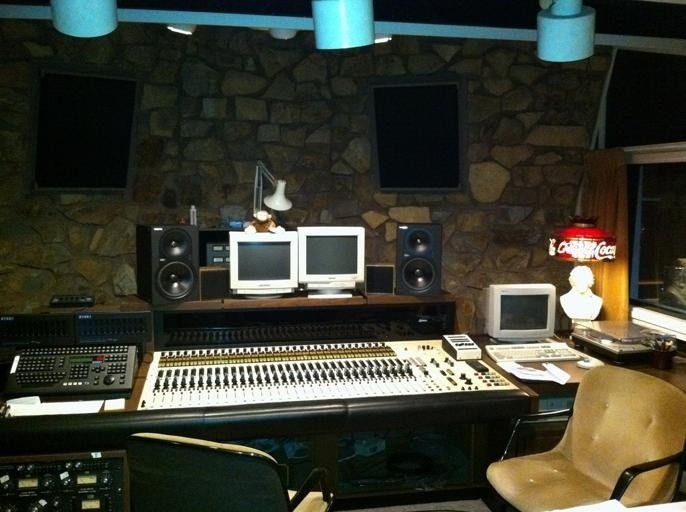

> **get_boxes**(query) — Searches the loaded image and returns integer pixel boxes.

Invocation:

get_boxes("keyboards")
[485,342,580,363]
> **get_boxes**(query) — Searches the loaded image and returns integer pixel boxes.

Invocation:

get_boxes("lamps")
[545,216,617,320]
[254,160,292,212]
[0,0,686,62]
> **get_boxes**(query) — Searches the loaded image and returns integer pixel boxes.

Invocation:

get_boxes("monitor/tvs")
[228,231,299,298]
[297,226,365,300]
[481,283,556,344]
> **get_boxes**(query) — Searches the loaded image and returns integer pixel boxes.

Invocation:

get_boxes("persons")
[559,266,603,320]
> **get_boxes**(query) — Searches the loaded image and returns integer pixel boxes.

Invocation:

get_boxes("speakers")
[395,223,442,296]
[136,223,200,306]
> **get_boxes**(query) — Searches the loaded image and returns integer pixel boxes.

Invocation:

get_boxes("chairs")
[128,432,334,511]
[486,365,686,512]
